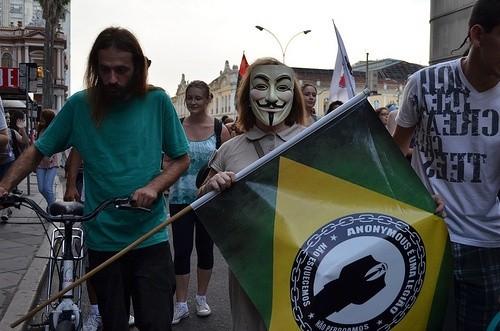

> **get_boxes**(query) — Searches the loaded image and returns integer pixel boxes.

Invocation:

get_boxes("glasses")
[380,113,389,116]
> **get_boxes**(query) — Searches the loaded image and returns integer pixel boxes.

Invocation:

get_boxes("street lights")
[256,25,312,65]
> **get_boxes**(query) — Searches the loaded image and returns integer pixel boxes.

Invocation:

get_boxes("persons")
[222,115,239,137]
[301,83,343,128]
[197,57,447,331]
[0,27,191,331]
[392,0,500,331]
[163,80,230,323]
[375,104,416,162]
[64,147,134,331]
[0,96,62,220]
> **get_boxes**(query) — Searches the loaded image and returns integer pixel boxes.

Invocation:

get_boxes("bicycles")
[0,190,154,331]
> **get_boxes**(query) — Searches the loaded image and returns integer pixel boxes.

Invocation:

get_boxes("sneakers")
[172,305,188,324]
[128,315,134,325]
[80,312,101,331]
[196,299,211,315]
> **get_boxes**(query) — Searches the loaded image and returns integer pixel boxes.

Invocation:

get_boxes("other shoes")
[0,208,12,220]
[10,187,25,197]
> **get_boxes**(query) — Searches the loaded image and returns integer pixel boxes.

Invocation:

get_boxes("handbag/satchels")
[11,129,21,159]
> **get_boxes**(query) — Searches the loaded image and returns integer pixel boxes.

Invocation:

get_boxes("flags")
[236,54,249,109]
[330,30,355,102]
[192,98,457,331]
